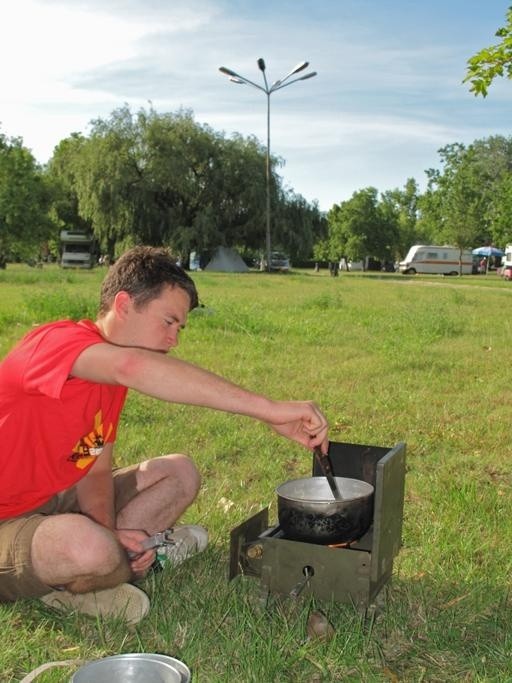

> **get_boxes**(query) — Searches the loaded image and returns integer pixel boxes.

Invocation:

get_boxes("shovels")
[304,568,335,642]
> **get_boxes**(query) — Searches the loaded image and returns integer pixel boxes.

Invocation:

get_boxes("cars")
[252,250,290,272]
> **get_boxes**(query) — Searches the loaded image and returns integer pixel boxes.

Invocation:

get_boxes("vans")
[397,244,473,277]
[338,257,363,272]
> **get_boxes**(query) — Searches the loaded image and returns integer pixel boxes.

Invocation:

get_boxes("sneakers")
[40,584,149,627]
[152,524,209,570]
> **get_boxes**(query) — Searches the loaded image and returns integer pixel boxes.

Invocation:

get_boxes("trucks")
[58,230,95,269]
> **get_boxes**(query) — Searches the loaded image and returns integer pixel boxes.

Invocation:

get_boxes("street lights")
[216,57,316,270]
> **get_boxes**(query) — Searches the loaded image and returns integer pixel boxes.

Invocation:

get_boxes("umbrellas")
[471,244,506,275]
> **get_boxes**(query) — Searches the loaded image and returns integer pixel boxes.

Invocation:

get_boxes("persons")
[0,242,334,627]
[477,255,487,272]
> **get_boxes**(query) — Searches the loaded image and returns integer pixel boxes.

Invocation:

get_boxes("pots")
[277,476,375,546]
